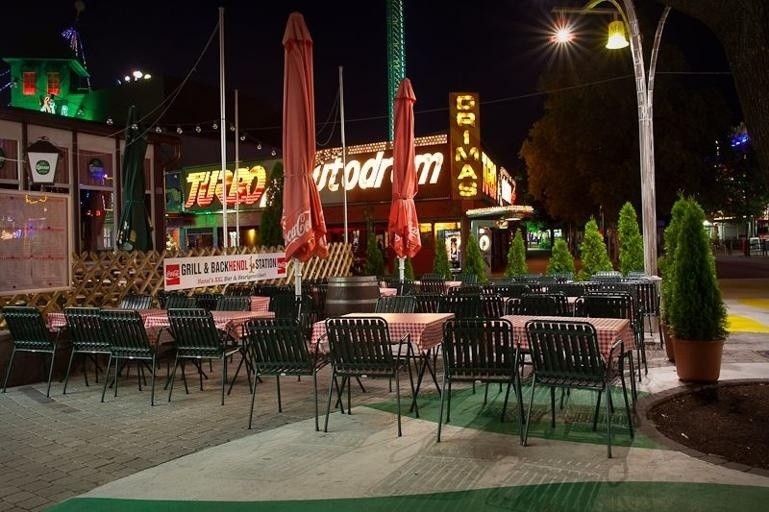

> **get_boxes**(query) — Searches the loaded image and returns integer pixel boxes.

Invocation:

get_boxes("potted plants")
[659,188,730,383]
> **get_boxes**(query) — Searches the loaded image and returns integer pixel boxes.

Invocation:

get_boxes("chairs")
[166,306,250,407]
[322,316,423,437]
[239,314,346,432]
[432,316,526,446]
[116,272,659,383]
[522,319,638,458]
[98,309,178,407]
[58,305,149,394]
[1,303,91,396]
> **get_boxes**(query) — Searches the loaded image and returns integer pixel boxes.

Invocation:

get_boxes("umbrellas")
[384,75,424,299]
[115,101,156,254]
[281,12,331,327]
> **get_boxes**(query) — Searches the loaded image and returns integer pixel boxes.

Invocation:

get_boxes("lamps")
[25,140,61,192]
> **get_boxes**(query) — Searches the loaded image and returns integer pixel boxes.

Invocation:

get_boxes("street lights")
[551,0,672,333]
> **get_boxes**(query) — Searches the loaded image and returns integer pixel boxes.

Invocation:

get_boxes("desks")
[144,309,276,395]
[45,309,167,385]
[480,313,637,431]
[311,311,455,411]
